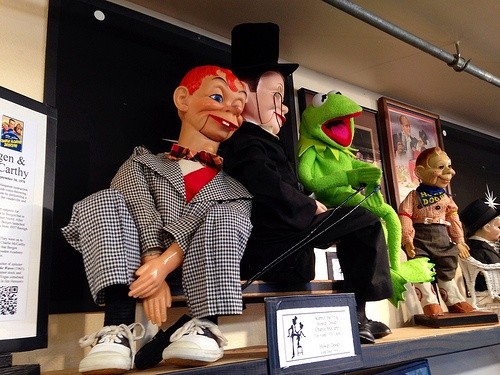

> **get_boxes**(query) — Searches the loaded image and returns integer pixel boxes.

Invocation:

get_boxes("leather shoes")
[358,317,392,344]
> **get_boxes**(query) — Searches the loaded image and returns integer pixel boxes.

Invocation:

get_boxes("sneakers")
[162,318,229,368]
[78,322,145,375]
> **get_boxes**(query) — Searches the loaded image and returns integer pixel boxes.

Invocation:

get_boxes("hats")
[230,22,299,79]
[459,199,500,237]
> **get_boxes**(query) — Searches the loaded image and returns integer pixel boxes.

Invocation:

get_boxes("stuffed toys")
[295,91,436,304]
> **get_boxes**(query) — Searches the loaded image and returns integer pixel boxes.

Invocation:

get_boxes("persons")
[397,147,476,318]
[459,199,500,291]
[218,23,393,348]
[63,65,255,375]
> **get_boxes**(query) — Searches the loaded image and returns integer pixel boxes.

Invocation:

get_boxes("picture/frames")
[379,95,453,212]
[297,86,392,207]
